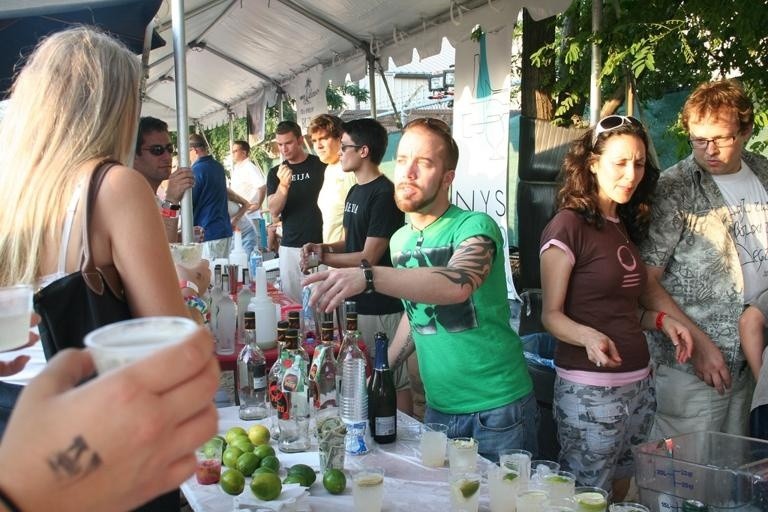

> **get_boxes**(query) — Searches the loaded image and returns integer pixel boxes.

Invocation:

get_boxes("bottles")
[209,265,223,331]
[268,321,300,442]
[238,270,254,344]
[215,274,235,355]
[247,267,278,350]
[312,322,341,414]
[278,329,311,453]
[288,311,310,363]
[368,332,397,443]
[229,226,247,282]
[343,332,366,424]
[250,247,263,281]
[337,302,367,403]
[237,312,268,420]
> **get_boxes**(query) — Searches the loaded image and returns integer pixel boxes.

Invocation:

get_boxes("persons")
[0,324,220,512]
[301,117,415,416]
[638,85,768,479]
[301,118,538,466]
[230,139,266,229]
[267,121,328,306]
[309,113,358,272]
[539,116,693,502]
[188,132,233,261]
[227,184,260,260]
[0,290,40,379]
[738,285,768,459]
[0,23,212,442]
[132,115,194,245]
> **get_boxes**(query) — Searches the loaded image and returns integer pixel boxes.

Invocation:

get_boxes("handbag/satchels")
[30,156,131,364]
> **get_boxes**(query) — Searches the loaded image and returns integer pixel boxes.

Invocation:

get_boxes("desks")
[175,394,532,512]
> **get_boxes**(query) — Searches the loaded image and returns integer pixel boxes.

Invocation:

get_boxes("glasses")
[591,114,641,148]
[687,136,736,150]
[142,143,174,156]
[339,140,362,151]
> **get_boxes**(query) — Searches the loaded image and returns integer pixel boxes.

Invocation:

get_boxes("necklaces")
[407,203,453,251]
[598,213,632,245]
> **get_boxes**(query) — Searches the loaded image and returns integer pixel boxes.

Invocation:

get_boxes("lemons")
[201,436,228,459]
[260,455,280,473]
[226,426,246,444]
[459,479,479,498]
[283,474,307,487]
[221,469,245,496]
[502,472,517,483]
[222,446,246,467]
[573,492,605,510]
[288,463,316,487]
[323,468,347,494]
[247,423,270,445]
[252,467,277,477]
[250,473,281,502]
[232,436,254,454]
[234,452,259,477]
[547,475,569,486]
[256,445,275,460]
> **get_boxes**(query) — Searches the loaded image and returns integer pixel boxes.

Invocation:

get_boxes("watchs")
[359,257,377,295]
[654,308,668,332]
[160,199,183,221]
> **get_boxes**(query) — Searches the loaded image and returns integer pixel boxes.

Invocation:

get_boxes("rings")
[595,358,602,369]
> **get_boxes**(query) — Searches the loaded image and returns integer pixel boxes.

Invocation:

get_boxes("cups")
[609,502,648,512]
[304,252,318,274]
[525,460,560,492]
[421,423,449,469]
[0,286,34,352]
[174,243,204,270]
[487,462,520,511]
[194,438,223,485]
[499,449,532,490]
[569,486,609,512]
[319,432,346,474]
[350,467,386,512]
[515,488,551,512]
[538,471,576,510]
[339,358,370,456]
[193,226,203,241]
[449,468,481,511]
[84,316,197,376]
[448,437,479,478]
